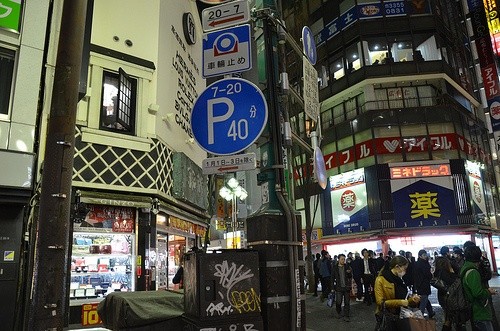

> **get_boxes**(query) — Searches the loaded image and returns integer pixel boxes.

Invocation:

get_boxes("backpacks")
[319,260,329,277]
[478,256,492,280]
[444,267,481,323]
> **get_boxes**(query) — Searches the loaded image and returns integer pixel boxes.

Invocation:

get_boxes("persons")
[305,241,500,331]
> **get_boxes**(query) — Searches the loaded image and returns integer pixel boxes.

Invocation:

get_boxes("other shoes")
[343,317,350,321]
[429,312,435,319]
[337,313,340,319]
[356,297,364,302]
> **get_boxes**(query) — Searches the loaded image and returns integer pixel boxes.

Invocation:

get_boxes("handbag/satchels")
[327,293,335,307]
[400,292,424,320]
[430,278,448,291]
[376,300,411,331]
